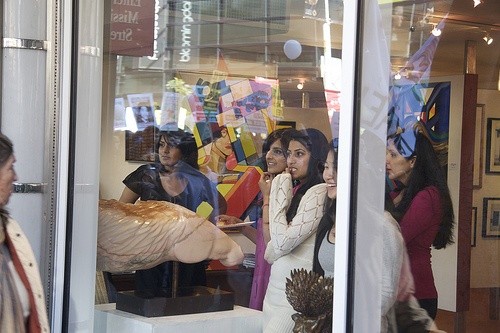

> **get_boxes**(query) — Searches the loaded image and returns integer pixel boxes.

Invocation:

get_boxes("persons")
[119,121,228,296]
[246,126,298,313]
[1,132,52,333]
[313,135,405,333]
[262,128,330,332]
[386,128,457,321]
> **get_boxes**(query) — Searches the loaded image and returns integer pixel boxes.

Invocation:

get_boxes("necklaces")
[331,226,337,238]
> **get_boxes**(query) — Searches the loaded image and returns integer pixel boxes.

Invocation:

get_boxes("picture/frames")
[482,197,500,237]
[470,205,477,247]
[473,103,485,189]
[485,117,500,175]
